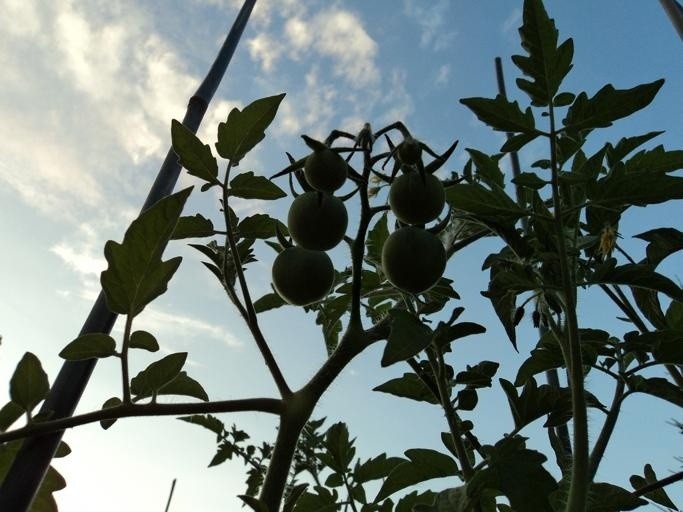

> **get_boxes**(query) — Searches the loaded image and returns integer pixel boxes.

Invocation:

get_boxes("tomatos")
[303,147,350,192]
[270,244,335,308]
[387,170,447,225]
[379,225,448,295]
[286,190,350,252]
[397,137,425,165]
